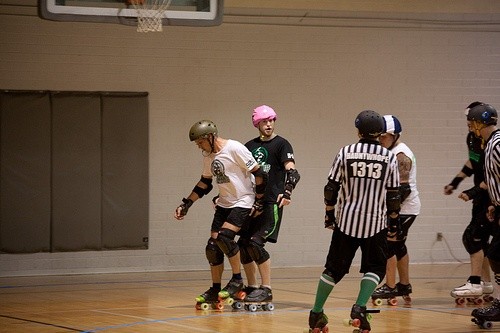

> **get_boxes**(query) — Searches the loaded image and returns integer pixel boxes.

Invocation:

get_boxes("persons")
[173,120,264,311]
[238,105,300,311]
[371,114,421,307]
[469,104,500,329]
[308,111,402,333]
[444,102,494,305]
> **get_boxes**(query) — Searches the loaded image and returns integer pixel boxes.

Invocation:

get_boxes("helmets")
[252,105,277,126]
[465,102,498,125]
[355,110,385,138]
[188,120,218,142]
[383,114,402,136]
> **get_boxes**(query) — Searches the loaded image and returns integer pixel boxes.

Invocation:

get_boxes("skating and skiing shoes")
[396,282,412,303]
[195,287,224,311]
[218,278,243,310]
[470,298,500,328]
[450,278,494,304]
[348,304,371,333]
[232,285,275,312]
[371,283,399,306]
[308,310,329,333]
